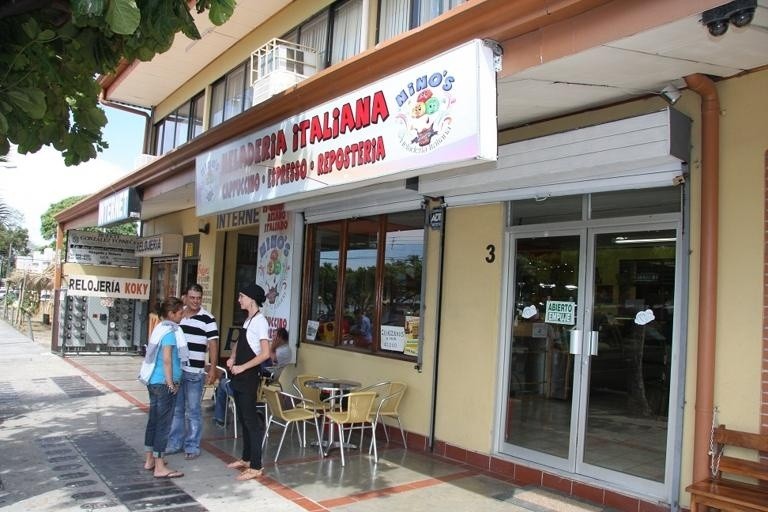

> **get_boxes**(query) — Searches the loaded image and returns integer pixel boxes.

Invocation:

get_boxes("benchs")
[685,425,767,512]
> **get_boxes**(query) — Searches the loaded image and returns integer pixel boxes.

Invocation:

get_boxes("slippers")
[154,470,184,478]
[144,458,168,471]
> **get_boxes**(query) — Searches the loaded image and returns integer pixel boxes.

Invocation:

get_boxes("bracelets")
[168,383,175,391]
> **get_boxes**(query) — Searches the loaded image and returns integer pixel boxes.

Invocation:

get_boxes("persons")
[522,300,562,432]
[143,297,190,478]
[210,327,293,427]
[350,309,373,341]
[226,280,271,481]
[164,283,219,461]
[381,302,389,326]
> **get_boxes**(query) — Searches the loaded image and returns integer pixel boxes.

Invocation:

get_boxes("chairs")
[200,360,408,465]
[511,294,676,420]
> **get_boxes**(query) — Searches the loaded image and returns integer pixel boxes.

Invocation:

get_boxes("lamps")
[196,217,210,236]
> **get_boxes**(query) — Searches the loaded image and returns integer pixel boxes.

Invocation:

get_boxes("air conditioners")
[256,46,319,77]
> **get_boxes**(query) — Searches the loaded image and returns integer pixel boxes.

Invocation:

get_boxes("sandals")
[235,467,264,480]
[226,460,251,469]
